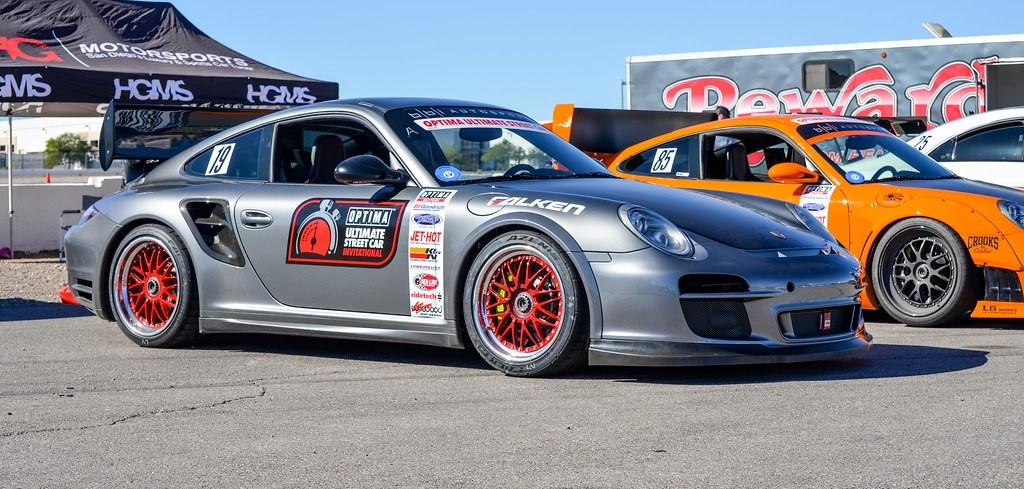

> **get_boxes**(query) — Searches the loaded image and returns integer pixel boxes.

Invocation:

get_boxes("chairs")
[60,195,102,259]
[305,135,345,184]
[411,139,436,169]
[726,144,763,183]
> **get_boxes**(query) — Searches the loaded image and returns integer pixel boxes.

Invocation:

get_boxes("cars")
[606,106,1024,326]
[64,96,873,377]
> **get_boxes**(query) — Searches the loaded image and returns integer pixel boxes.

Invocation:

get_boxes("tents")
[0,1,341,260]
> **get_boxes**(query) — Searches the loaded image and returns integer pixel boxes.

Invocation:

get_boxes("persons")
[712,106,736,152]
